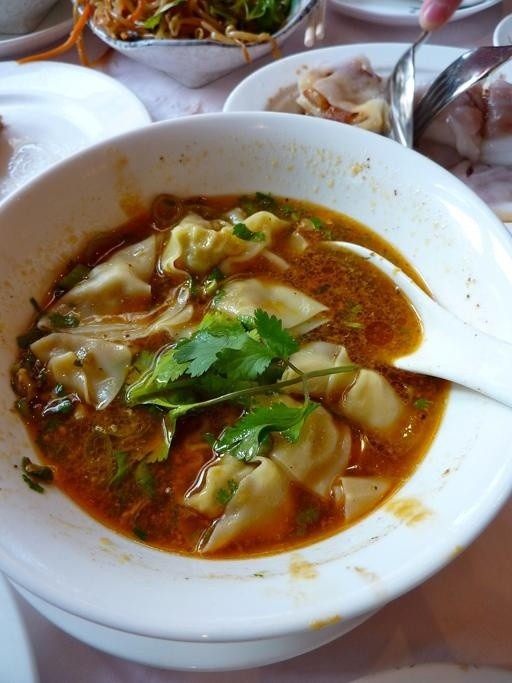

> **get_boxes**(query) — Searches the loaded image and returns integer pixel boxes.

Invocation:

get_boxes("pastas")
[88,1,285,61]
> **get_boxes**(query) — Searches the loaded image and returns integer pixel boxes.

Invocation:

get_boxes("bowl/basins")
[2,110,510,642]
[76,0,321,90]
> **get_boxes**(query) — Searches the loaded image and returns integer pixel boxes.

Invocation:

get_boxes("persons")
[418,1,465,31]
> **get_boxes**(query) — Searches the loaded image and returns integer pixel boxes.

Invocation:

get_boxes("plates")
[1,0,77,55]
[327,0,501,27]
[10,577,381,673]
[2,59,152,203]
[494,10,512,45]
[223,43,511,222]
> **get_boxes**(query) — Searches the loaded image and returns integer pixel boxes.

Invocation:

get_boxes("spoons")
[383,32,437,147]
[311,240,510,406]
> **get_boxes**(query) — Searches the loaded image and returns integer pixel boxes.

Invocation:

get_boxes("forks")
[414,44,512,141]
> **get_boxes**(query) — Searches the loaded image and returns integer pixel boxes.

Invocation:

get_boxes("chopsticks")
[303,0,330,46]
